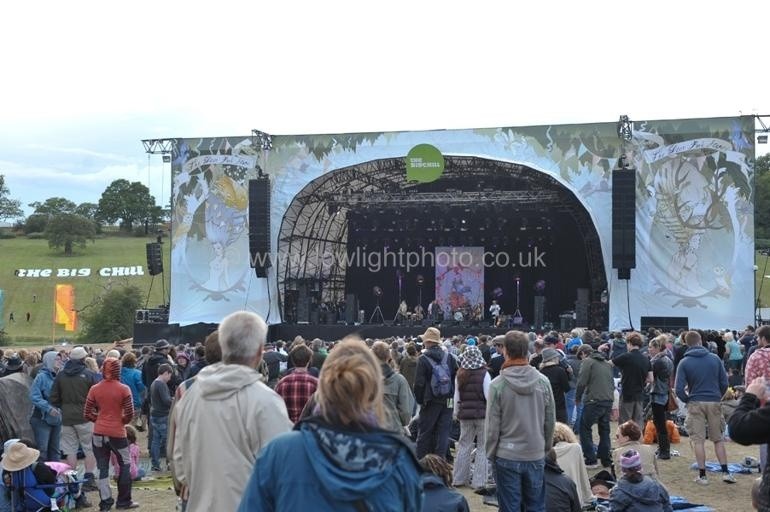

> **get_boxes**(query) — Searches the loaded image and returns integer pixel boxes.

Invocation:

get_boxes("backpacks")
[421,352,452,397]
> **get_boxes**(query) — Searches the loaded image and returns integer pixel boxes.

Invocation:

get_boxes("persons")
[200,243,232,292]
[474,303,483,321]
[488,300,501,327]
[399,299,441,322]
[1,309,769,511]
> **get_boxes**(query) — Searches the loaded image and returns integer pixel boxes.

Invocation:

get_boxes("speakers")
[145,241,163,275]
[610,168,636,281]
[248,178,271,279]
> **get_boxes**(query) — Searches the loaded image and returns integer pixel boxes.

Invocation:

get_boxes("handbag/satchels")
[665,389,679,411]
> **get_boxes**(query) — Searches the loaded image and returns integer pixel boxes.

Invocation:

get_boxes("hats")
[5,353,25,370]
[176,353,189,362]
[155,339,169,349]
[420,328,595,369]
[69,347,87,360]
[1,438,40,472]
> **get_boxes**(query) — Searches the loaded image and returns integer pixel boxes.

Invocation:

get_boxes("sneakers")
[71,480,139,511]
[585,446,737,486]
